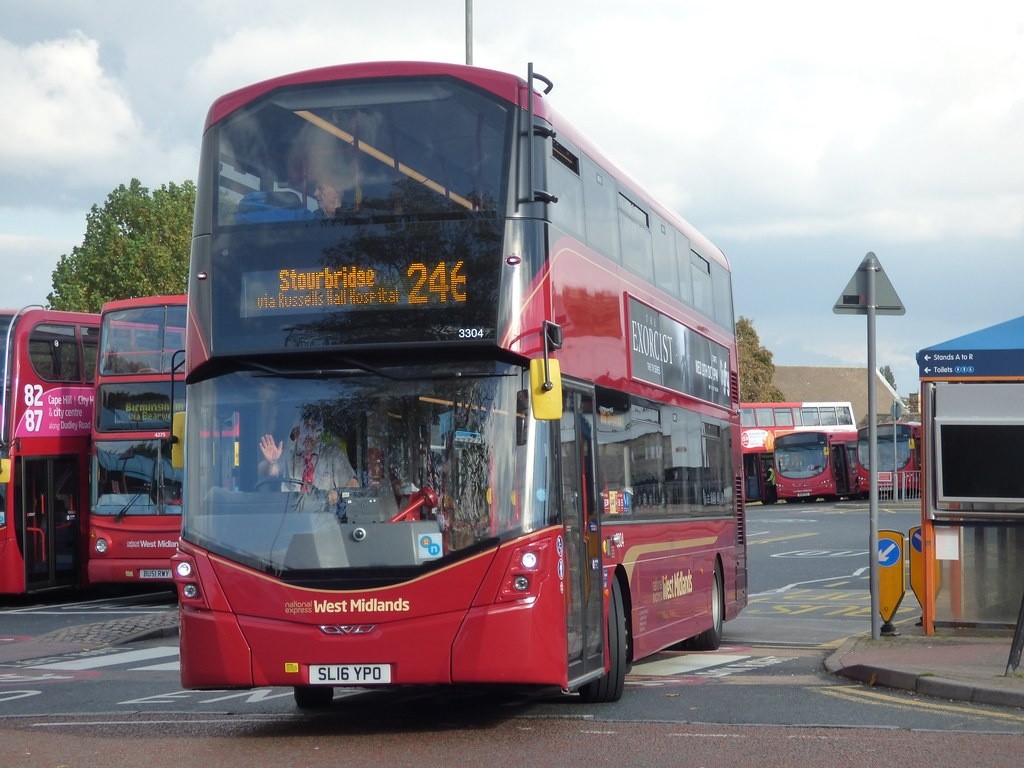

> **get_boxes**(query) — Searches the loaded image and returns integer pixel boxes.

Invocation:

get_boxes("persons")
[312,170,345,217]
[258,413,359,506]
[766,468,776,504]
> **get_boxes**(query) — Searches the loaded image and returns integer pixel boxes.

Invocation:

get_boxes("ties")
[300,450,315,493]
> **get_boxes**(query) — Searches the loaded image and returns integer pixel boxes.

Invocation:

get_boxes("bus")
[171,61,749,710]
[0,304,102,604]
[739,402,856,501]
[856,419,920,496]
[85,294,189,584]
[773,430,858,503]
